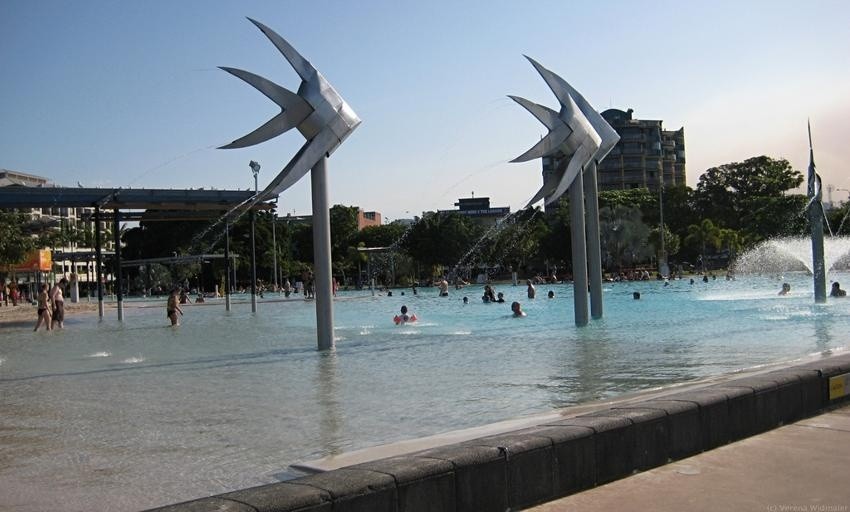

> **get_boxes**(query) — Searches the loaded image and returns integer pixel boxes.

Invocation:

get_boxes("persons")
[237,269,316,299]
[779,283,790,296]
[143,285,205,325]
[604,264,737,299]
[830,283,847,297]
[333,273,558,324]
[1,278,68,331]
